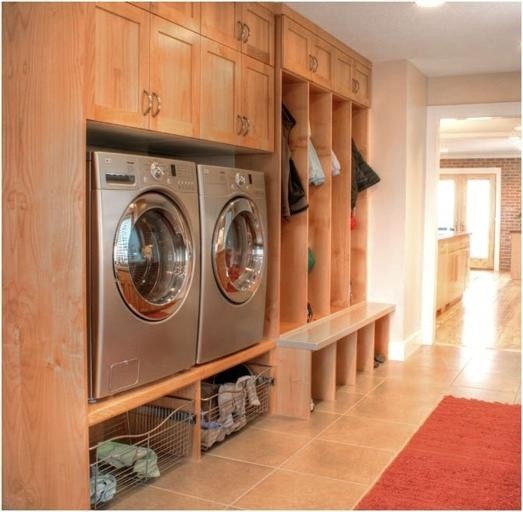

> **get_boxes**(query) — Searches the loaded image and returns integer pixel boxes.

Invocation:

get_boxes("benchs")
[275,301,395,422]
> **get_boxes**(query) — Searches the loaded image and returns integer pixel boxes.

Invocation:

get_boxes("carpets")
[353,395,522,511]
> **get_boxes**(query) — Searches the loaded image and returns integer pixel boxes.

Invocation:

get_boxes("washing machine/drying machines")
[196,163,268,368]
[86,148,202,400]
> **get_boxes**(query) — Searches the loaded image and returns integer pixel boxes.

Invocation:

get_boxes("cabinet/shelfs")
[274,3,372,110]
[84,2,277,155]
[437,236,470,316]
[234,69,368,415]
